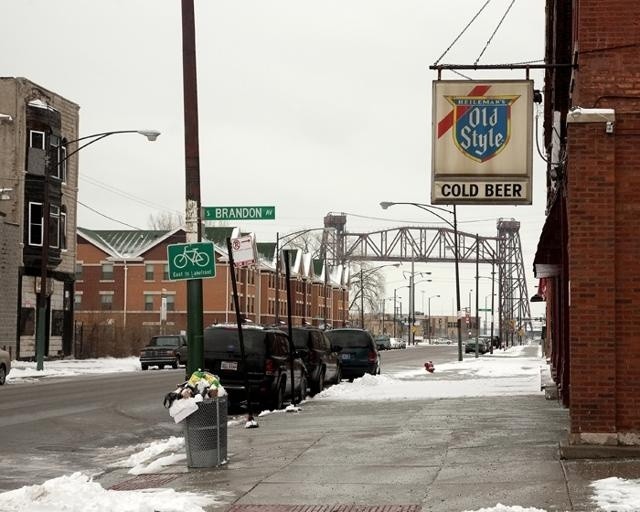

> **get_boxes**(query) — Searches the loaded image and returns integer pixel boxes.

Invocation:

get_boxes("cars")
[140,335,188,370]
[324,329,380,382]
[374,335,406,350]
[465,335,498,353]
[434,338,453,345]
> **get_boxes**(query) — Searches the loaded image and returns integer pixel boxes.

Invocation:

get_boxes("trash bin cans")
[182,392,227,468]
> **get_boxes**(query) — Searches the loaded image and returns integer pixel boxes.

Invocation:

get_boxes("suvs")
[203,323,309,412]
[263,327,343,397]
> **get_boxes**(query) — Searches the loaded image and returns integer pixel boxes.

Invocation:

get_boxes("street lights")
[389,285,411,338]
[275,227,336,324]
[410,272,432,343]
[445,245,502,349]
[348,263,400,289]
[380,201,462,362]
[36,125,160,371]
